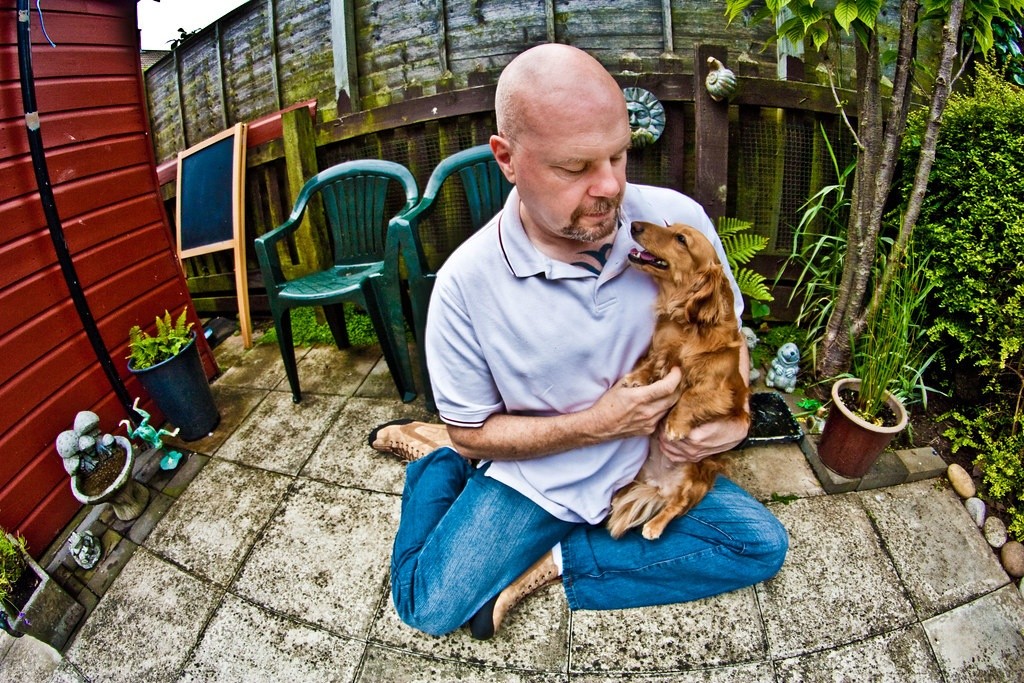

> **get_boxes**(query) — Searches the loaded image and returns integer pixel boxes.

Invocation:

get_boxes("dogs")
[605,220,755,543]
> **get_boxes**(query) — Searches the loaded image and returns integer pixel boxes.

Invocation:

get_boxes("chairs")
[394,142,516,412]
[253,158,420,406]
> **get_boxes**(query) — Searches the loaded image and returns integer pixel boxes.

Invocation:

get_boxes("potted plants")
[123,305,223,444]
[816,205,939,480]
[53,409,151,523]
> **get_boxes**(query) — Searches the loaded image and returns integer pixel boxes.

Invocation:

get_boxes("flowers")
[0,526,35,630]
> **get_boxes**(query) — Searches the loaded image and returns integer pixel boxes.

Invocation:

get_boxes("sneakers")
[367,421,456,463]
[470,550,558,639]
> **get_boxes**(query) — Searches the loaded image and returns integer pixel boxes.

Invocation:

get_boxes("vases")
[4,549,86,652]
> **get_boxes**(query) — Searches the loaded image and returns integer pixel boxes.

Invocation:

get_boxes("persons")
[365,42,788,642]
